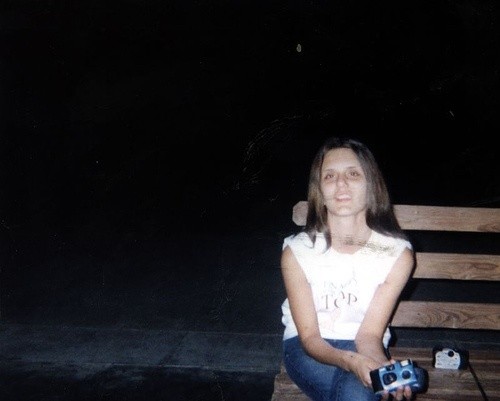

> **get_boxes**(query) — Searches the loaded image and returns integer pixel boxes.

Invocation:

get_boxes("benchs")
[271,201,500,401]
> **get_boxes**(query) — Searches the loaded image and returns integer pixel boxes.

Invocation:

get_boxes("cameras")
[370,359,418,399]
[433,345,470,370]
